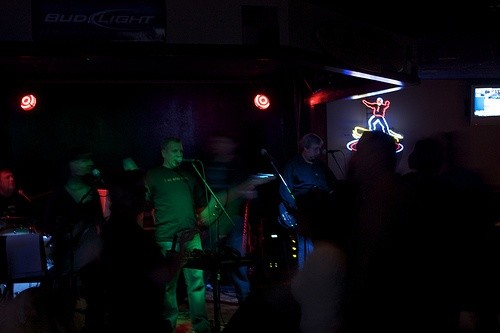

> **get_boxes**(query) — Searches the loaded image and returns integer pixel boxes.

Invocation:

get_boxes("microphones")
[92,169,104,184]
[323,150,339,154]
[176,157,195,164]
[261,149,276,161]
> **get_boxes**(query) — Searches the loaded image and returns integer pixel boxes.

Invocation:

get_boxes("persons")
[57,138,99,243]
[0,167,27,227]
[289,186,351,332]
[349,133,500,333]
[143,136,214,333]
[278,133,331,274]
[1,213,189,332]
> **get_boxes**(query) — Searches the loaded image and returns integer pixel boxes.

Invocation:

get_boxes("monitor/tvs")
[470,84,500,126]
[10,279,42,301]
